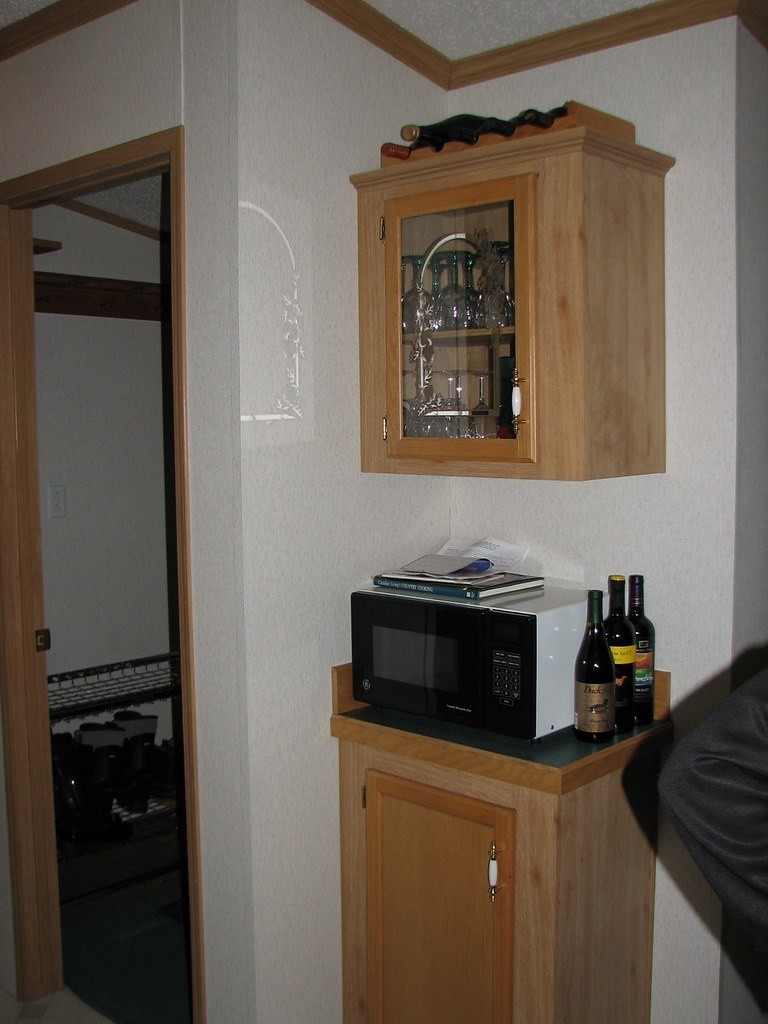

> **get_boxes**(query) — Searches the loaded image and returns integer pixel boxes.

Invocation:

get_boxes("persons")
[655,657,767,969]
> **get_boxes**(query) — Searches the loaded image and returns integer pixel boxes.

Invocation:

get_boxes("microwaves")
[350,559,610,741]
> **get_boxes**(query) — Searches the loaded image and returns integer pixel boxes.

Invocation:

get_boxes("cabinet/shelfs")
[350,126,677,480]
[46,649,187,906]
[329,705,662,1023]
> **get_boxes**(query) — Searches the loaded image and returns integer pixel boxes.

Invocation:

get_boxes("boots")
[74,711,158,814]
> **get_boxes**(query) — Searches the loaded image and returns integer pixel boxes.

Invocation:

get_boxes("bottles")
[626,575,655,728]
[574,590,616,744]
[603,575,636,737]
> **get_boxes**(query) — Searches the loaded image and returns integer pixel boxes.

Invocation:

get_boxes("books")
[375,570,550,603]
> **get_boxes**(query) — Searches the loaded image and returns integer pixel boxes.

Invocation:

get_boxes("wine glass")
[402,258,432,334]
[474,244,515,329]
[425,264,448,331]
[458,253,478,306]
[435,252,474,330]
